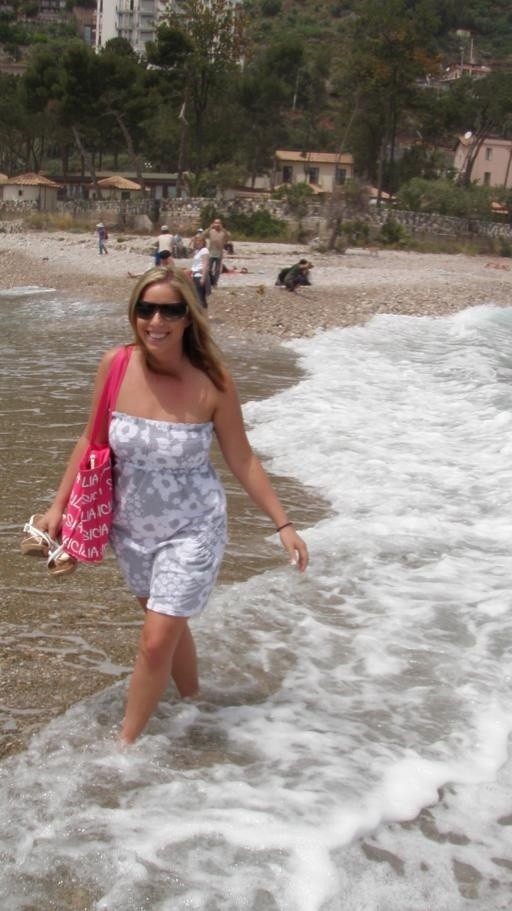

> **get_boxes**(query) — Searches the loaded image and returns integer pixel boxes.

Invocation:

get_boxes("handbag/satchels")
[59,345,135,564]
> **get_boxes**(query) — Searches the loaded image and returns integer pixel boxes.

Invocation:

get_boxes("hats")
[161,224,169,232]
[95,222,104,228]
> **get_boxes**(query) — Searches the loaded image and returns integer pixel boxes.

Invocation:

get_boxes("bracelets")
[275,521,294,531]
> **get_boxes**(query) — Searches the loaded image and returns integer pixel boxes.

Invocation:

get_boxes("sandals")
[20,512,52,559]
[45,536,77,577]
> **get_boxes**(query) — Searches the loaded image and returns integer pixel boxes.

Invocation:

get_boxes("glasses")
[134,299,190,322]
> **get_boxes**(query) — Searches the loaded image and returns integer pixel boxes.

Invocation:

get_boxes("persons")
[95,221,109,255]
[35,264,312,747]
[150,217,237,309]
[283,260,315,291]
